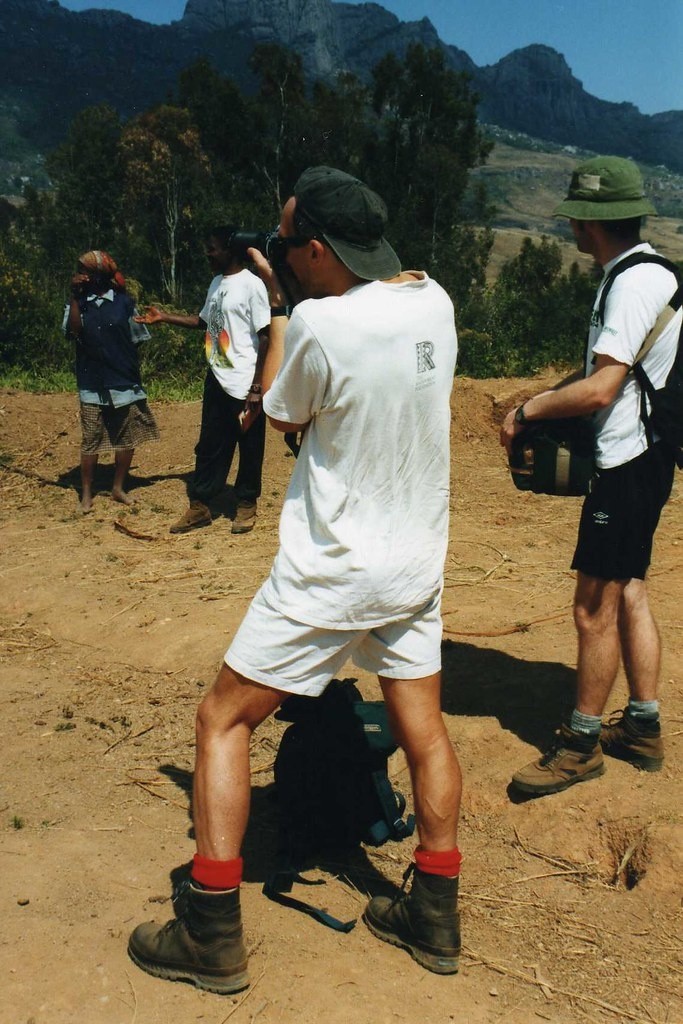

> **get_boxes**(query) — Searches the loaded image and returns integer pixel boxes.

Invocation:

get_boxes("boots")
[170,499,212,533]
[232,499,257,533]
[127,880,250,993]
[366,865,461,973]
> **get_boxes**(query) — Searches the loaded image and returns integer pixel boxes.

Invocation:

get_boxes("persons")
[501,156,683,794]
[60,250,160,515]
[132,226,266,534]
[128,166,463,994]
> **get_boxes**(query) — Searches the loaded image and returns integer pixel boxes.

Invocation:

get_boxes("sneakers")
[600,707,664,772]
[511,724,607,794]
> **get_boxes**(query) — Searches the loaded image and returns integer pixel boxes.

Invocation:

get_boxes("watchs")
[515,405,529,425]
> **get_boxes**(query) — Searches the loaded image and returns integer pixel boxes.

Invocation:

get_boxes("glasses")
[274,237,309,258]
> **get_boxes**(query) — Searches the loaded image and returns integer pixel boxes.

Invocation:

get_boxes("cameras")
[224,223,292,277]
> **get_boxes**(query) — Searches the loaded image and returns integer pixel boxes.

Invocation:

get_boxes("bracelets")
[271,305,294,317]
[249,384,261,394]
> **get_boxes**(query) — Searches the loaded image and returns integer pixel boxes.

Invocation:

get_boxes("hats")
[553,155,658,221]
[294,166,401,280]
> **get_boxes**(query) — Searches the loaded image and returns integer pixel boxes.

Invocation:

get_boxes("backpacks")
[600,251,683,465]
[274,677,412,864]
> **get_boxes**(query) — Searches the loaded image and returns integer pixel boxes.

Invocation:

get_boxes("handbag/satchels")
[505,415,594,495]
